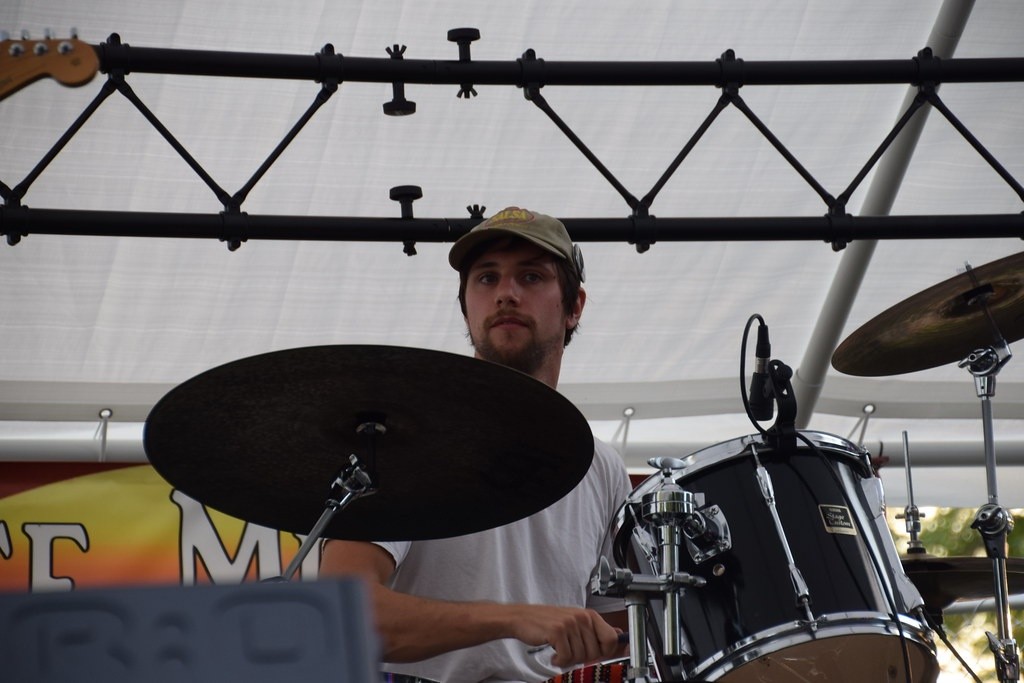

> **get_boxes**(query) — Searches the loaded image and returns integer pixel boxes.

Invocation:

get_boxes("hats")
[448,208,579,278]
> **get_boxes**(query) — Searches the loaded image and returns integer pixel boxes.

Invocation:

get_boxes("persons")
[312,206,654,683]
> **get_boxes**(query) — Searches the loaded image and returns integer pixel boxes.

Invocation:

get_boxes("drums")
[611,425,941,682]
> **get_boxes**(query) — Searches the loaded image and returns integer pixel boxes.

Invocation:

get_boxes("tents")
[2,0,1024,523]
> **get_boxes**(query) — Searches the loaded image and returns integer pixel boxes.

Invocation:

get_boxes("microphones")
[748,324,774,421]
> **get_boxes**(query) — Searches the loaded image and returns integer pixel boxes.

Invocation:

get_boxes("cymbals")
[144,342,597,543]
[900,553,1024,601]
[831,251,1024,378]
